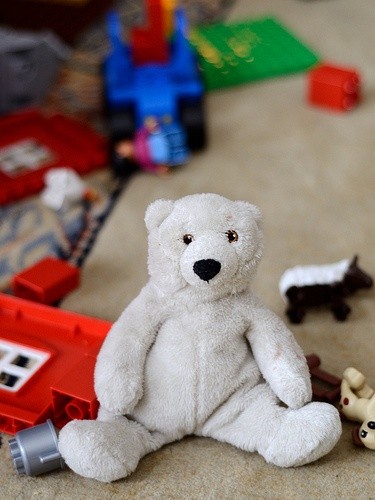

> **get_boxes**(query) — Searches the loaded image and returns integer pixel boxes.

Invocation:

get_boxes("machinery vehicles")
[100,0,209,181]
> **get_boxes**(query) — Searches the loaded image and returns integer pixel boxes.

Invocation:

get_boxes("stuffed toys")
[59,193,344,482]
[339,367,375,450]
[279,252,374,325]
